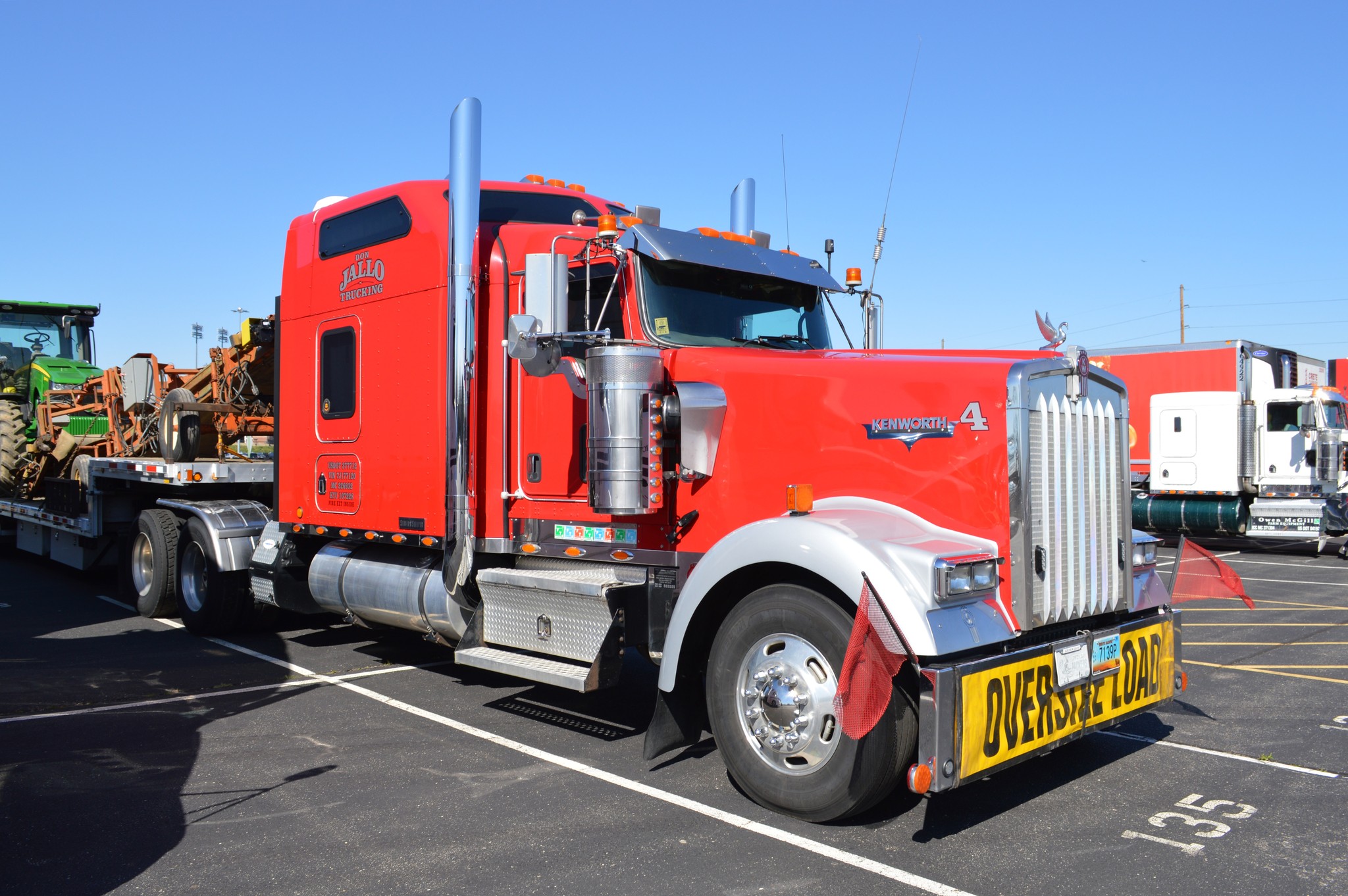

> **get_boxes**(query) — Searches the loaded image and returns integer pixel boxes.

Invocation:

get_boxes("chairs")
[12,348,32,369]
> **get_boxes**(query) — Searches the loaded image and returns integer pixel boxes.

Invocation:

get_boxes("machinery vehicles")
[0,296,125,497]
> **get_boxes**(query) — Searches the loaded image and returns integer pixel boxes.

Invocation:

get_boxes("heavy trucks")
[1058,340,1348,561]
[1,99,1189,825]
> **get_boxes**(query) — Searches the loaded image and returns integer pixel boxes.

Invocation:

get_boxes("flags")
[1172,538,1256,610]
[831,578,914,740]
